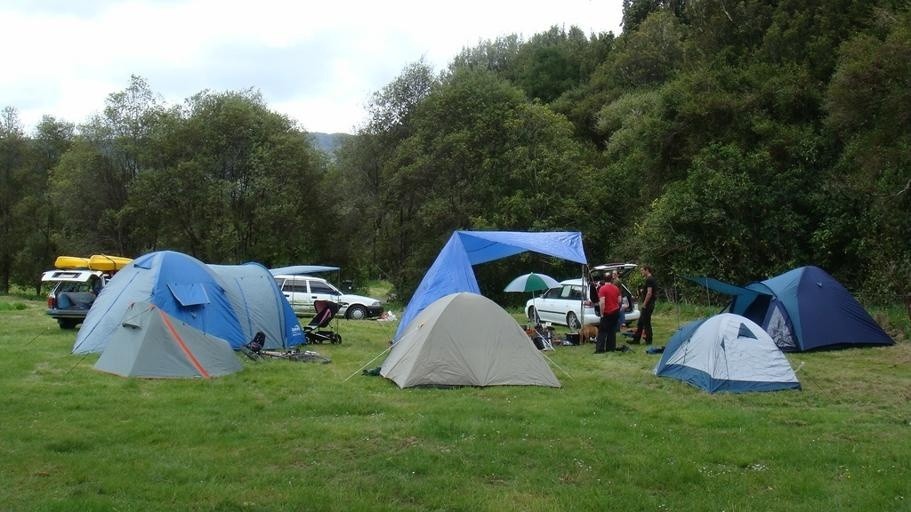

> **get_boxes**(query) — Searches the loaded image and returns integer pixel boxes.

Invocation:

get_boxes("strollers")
[301,300,342,346]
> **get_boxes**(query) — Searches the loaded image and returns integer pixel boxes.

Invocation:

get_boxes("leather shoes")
[626,335,640,344]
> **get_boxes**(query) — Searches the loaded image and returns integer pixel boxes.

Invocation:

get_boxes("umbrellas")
[503,272,563,333]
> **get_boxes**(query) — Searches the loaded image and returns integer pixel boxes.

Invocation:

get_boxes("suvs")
[41,269,114,330]
[525,262,641,331]
[275,274,384,321]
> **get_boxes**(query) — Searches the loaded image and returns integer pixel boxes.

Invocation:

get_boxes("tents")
[268,261,342,343]
[71,250,308,355]
[377,291,562,389]
[387,229,592,346]
[673,265,897,354]
[651,313,803,396]
[92,302,244,379]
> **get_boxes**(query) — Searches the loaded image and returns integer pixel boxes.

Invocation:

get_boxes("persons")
[612,270,622,289]
[593,272,621,354]
[626,265,656,345]
